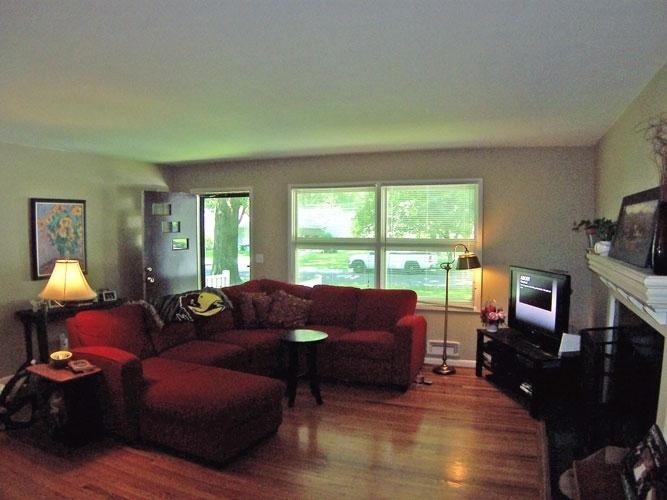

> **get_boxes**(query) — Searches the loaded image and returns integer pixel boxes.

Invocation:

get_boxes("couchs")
[64,277,427,472]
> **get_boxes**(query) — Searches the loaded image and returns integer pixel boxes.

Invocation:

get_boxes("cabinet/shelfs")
[476,328,578,421]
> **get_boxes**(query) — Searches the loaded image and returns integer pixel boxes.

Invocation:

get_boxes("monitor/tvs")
[508,265,570,357]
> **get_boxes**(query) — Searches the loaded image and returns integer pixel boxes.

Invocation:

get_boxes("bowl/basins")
[50,350,72,368]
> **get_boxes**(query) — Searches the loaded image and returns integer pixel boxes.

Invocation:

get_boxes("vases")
[585,227,611,249]
[486,322,499,333]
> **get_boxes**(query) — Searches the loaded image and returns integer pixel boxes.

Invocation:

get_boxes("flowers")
[479,299,507,322]
[571,217,617,235]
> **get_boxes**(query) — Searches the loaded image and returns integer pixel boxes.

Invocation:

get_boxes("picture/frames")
[29,197,88,280]
[608,184,665,268]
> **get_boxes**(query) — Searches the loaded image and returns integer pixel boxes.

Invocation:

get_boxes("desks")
[277,327,327,409]
[14,299,122,363]
[24,360,101,451]
[572,458,630,500]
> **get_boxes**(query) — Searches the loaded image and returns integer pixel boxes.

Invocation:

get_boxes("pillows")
[264,291,313,328]
[238,289,266,329]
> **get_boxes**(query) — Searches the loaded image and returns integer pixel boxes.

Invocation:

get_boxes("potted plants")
[632,111,667,275]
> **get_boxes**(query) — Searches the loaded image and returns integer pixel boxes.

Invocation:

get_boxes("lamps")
[37,259,97,303]
[432,243,481,375]
